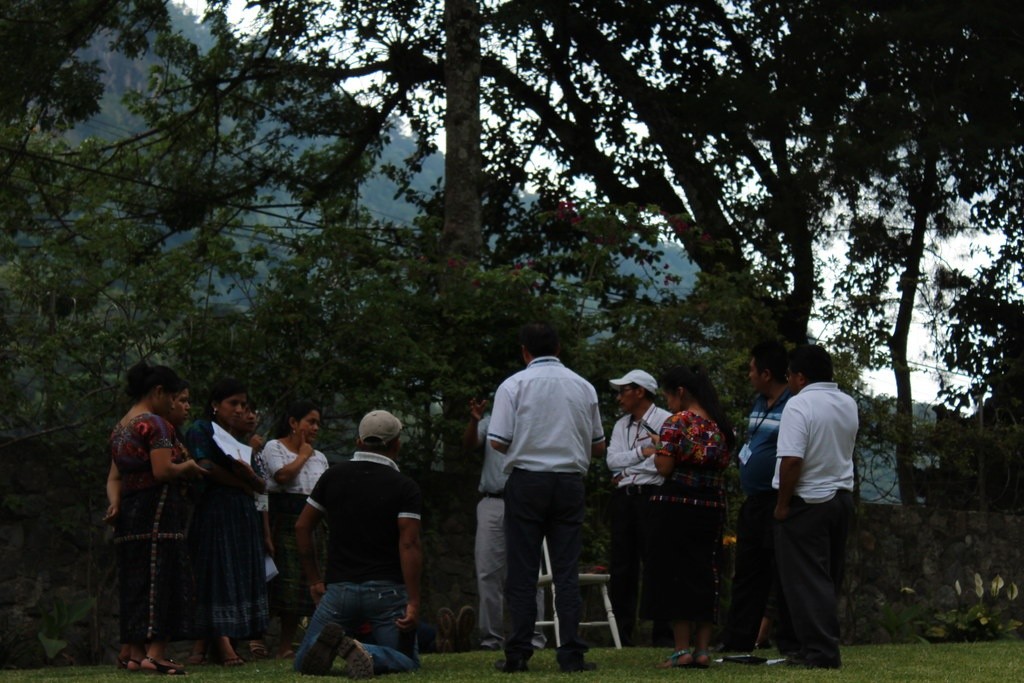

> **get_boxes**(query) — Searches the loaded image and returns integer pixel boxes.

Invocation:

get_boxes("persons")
[710,340,801,654]
[488,324,606,675]
[607,370,679,647]
[647,366,730,670]
[465,398,549,654]
[295,410,424,680]
[184,379,270,668]
[261,402,330,661]
[772,343,859,672]
[103,364,208,676]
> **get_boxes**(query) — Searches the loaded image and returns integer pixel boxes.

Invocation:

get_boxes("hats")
[609,369,658,395]
[359,410,402,446]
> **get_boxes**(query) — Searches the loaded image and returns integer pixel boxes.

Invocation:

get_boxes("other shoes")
[479,636,500,650]
[495,655,527,673]
[609,626,635,647]
[532,638,545,649]
[436,608,455,653]
[301,622,345,676]
[652,631,676,647]
[561,655,597,673]
[455,606,475,652]
[337,637,373,681]
[718,640,838,669]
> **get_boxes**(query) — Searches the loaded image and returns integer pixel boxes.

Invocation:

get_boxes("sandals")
[116,641,296,675]
[654,651,692,668]
[692,652,712,668]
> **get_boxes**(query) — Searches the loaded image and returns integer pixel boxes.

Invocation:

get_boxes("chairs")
[535,533,621,649]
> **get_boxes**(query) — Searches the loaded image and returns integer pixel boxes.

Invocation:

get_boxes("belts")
[616,484,649,495]
[750,491,777,503]
[484,492,505,498]
[510,467,584,479]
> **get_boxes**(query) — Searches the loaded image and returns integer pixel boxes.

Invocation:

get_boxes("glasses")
[784,370,795,378]
[619,388,640,395]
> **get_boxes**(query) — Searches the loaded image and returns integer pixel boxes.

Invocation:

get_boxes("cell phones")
[200,463,214,469]
[641,424,658,435]
